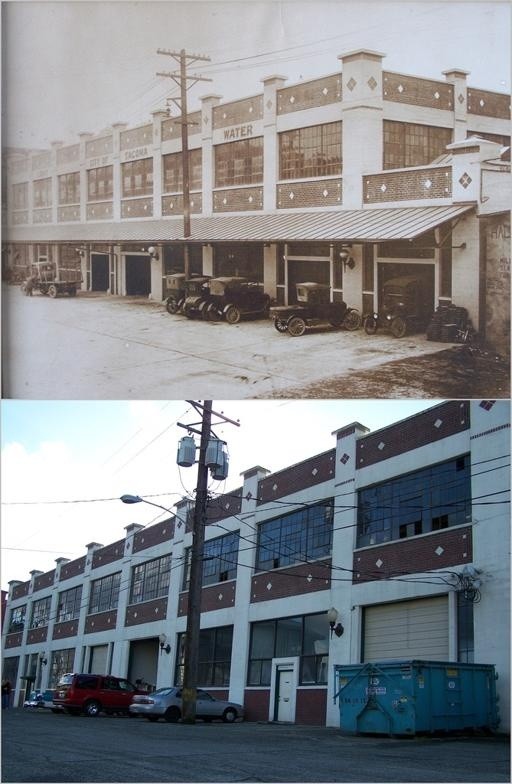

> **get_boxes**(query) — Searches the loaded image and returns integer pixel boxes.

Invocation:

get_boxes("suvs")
[53,671,150,720]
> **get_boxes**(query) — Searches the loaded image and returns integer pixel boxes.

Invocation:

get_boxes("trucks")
[22,688,69,712]
[19,260,84,299]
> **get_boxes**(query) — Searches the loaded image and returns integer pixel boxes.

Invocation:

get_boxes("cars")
[270,281,361,337]
[162,270,274,326]
[363,275,432,337]
[128,685,250,725]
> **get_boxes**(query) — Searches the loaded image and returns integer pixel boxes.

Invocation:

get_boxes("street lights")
[121,490,196,539]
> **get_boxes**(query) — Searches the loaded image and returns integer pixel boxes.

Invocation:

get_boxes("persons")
[1,676,12,710]
[26,273,37,296]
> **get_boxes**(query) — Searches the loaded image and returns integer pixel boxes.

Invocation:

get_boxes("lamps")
[326,607,344,639]
[147,246,160,260]
[75,248,84,256]
[39,653,47,665]
[339,250,355,270]
[158,633,171,656]
